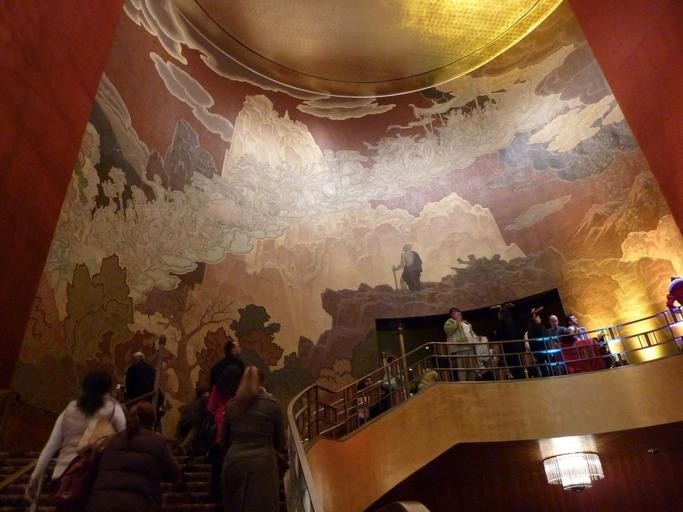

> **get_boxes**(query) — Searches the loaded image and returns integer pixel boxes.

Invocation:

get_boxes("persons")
[218,365,288,511]
[23,366,127,491]
[83,399,184,511]
[665,276,683,309]
[391,243,422,293]
[442,305,581,384]
[351,353,439,429]
[113,339,246,454]
[205,364,243,500]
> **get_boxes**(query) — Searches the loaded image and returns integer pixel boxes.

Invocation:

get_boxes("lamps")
[542,451,605,493]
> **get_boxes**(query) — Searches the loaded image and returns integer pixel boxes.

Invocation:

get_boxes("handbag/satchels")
[47,435,109,505]
[76,398,120,450]
